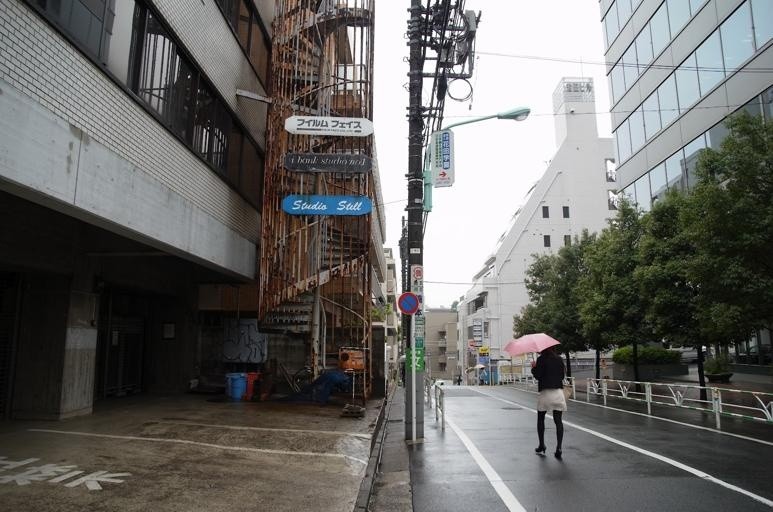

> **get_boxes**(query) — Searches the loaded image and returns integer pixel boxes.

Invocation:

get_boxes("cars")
[727,343,773,366]
[663,339,715,365]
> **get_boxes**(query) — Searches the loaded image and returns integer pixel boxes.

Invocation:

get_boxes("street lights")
[405,109,533,443]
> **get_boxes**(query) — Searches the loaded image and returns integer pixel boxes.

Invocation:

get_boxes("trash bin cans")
[224,372,259,400]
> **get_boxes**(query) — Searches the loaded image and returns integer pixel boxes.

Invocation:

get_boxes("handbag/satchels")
[563,386,572,403]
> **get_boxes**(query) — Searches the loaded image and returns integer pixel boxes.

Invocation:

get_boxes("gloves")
[532,361,536,367]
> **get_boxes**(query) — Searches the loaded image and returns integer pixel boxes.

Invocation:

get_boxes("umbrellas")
[472,363,486,369]
[504,332,561,360]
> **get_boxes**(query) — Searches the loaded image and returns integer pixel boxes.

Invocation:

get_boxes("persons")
[479,368,487,385]
[529,347,567,458]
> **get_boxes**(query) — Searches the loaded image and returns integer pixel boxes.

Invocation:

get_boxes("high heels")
[555,447,562,457]
[536,445,546,453]
